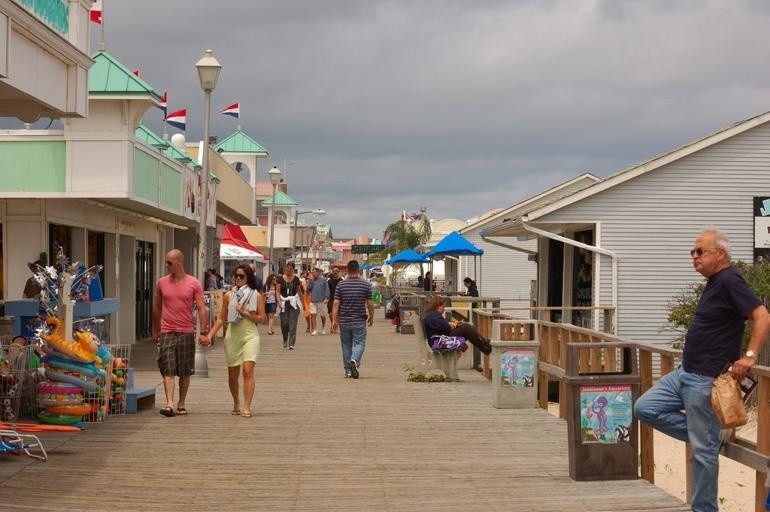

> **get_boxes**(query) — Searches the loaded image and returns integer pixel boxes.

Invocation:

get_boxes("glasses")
[165,261,175,266]
[235,274,244,279]
[272,277,276,280]
[690,247,719,256]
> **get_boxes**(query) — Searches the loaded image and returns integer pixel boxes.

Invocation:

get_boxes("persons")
[327,266,343,334]
[151,249,208,417]
[424,272,437,293]
[422,296,492,357]
[462,277,480,308]
[206,264,266,418]
[330,260,375,378]
[302,272,315,333]
[204,267,217,290]
[307,267,330,336]
[632,230,766,512]
[275,262,306,350]
[248,263,263,293]
[263,274,277,335]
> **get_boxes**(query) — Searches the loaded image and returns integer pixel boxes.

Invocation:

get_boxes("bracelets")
[200,331,209,336]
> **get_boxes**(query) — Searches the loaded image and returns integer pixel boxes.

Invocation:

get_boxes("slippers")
[242,412,252,417]
[231,409,241,415]
[159,406,174,416]
[176,408,187,415]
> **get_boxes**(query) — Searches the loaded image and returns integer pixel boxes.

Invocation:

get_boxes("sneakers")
[321,327,326,335]
[288,346,294,350]
[283,341,287,348]
[349,359,359,379]
[346,373,351,378]
[311,330,319,336]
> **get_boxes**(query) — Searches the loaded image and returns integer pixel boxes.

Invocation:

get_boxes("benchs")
[401,319,414,334]
[425,335,463,381]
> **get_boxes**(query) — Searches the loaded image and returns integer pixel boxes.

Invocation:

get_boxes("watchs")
[743,349,759,363]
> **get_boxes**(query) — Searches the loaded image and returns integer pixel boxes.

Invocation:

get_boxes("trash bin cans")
[561,341,642,482]
[490,319,541,410]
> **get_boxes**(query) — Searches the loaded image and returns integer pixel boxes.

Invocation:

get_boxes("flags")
[219,102,240,121]
[160,109,186,129]
[156,91,167,113]
[89,0,106,25]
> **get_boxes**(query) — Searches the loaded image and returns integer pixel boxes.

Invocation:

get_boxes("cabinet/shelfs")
[28,246,99,429]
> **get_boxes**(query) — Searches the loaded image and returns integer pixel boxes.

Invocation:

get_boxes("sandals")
[268,331,273,335]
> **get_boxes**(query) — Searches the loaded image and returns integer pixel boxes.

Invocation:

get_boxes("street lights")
[268,165,281,275]
[291,208,325,264]
[193,48,224,379]
